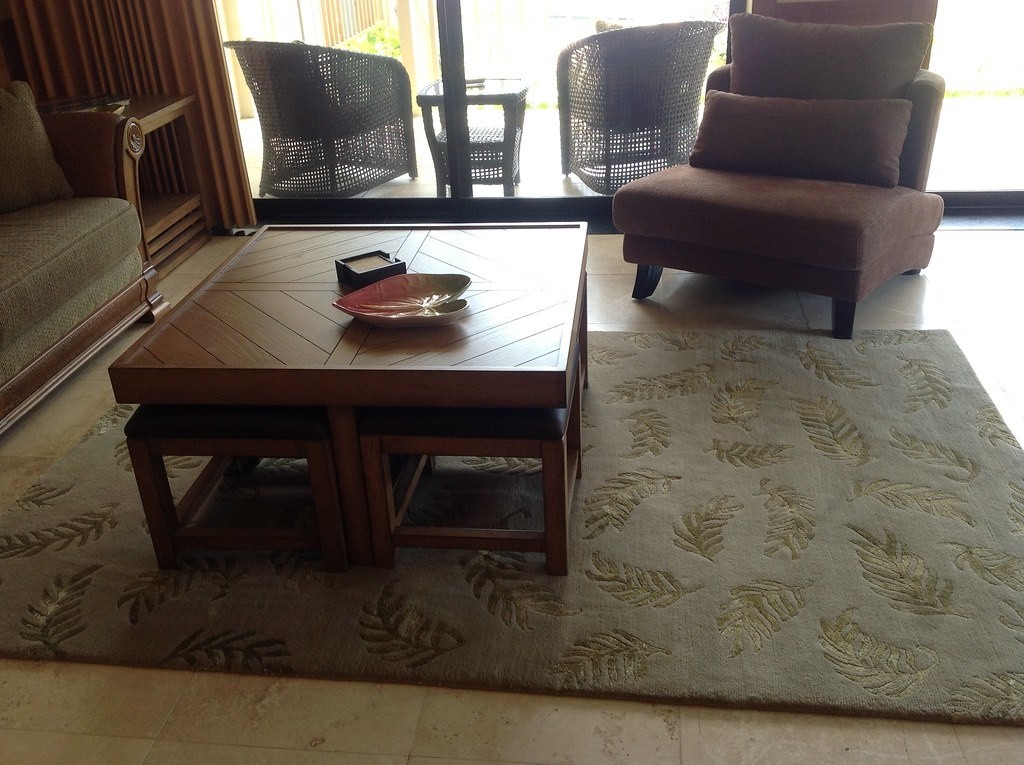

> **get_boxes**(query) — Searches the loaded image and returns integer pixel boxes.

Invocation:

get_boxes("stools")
[354,376,583,576]
[123,403,349,576]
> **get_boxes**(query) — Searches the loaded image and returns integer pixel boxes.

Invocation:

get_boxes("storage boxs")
[334,249,407,289]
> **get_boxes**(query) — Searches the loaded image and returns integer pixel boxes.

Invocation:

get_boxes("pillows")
[723,12,936,99]
[688,88,914,192]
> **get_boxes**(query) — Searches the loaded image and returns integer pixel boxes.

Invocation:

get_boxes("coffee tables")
[104,219,598,582]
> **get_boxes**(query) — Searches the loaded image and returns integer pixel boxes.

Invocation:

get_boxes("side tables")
[416,73,532,197]
[66,84,213,282]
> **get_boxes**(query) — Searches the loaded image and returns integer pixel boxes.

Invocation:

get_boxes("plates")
[332,274,472,327]
[55,103,126,114]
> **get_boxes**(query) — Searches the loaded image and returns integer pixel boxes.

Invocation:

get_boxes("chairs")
[555,20,728,195]
[223,37,420,197]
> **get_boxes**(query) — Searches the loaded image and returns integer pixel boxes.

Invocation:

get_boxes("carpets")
[0,331,1024,730]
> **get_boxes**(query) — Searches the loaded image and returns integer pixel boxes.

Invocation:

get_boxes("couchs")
[0,76,173,436]
[608,0,946,344]
